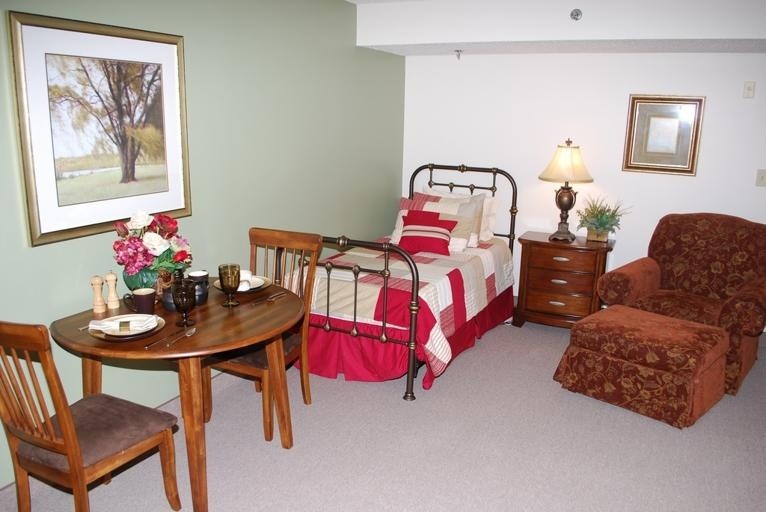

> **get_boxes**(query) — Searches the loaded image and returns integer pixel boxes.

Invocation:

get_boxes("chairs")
[597,211,765,396]
[199,225,326,442]
[0,319,182,511]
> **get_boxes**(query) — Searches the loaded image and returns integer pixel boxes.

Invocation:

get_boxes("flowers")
[109,209,194,275]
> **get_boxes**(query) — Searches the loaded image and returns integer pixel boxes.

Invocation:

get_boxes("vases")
[120,262,164,293]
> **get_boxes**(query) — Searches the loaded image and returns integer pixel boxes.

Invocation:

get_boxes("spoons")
[156,328,198,351]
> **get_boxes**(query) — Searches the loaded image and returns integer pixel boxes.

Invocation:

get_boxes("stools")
[554,303,731,428]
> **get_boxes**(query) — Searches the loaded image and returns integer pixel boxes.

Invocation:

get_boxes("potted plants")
[576,196,633,245]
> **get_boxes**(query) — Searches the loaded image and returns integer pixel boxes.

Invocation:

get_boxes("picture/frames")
[6,9,195,248]
[623,92,707,177]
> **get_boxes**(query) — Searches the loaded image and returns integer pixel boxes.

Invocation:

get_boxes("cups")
[122,288,156,314]
[240,271,251,284]
[162,287,177,311]
[187,269,208,305]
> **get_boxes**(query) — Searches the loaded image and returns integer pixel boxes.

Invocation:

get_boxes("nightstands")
[510,230,616,327]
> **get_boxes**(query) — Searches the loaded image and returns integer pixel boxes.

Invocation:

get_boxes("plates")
[211,275,273,292]
[87,314,166,342]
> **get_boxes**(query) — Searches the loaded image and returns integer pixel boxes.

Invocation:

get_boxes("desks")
[48,276,305,510]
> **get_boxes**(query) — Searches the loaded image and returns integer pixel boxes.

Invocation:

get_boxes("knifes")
[144,328,185,349]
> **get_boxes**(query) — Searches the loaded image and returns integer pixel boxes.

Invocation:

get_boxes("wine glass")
[170,278,196,329]
[218,262,240,308]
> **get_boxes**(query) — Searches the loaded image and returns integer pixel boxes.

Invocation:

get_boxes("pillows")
[388,187,499,258]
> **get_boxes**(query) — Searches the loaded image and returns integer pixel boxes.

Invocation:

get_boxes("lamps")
[539,138,593,244]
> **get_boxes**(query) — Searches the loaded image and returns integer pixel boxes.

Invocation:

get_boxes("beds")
[275,163,520,400]
